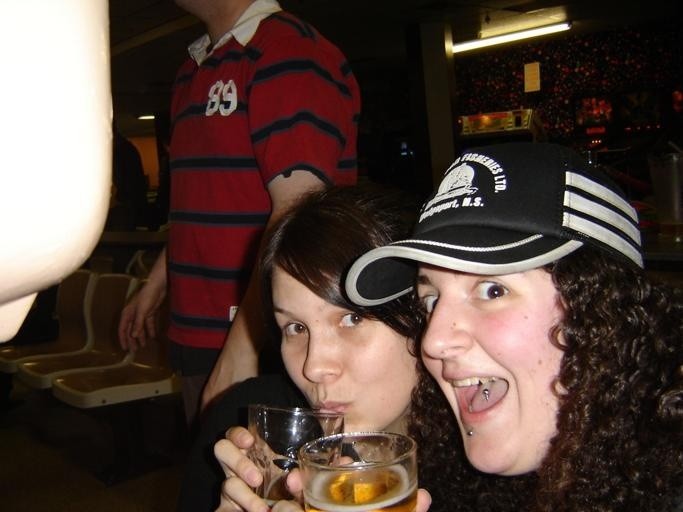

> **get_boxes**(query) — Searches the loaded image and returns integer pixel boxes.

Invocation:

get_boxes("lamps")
[450,5,573,54]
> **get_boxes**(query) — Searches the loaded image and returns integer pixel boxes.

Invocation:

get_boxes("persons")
[117,0,364,512]
[212,187,418,512]
[272,146,683,512]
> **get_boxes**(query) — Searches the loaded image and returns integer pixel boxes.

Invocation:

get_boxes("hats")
[343,146,643,308]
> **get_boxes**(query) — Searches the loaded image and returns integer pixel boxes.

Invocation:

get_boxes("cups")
[300,430,418,512]
[246,402,344,512]
